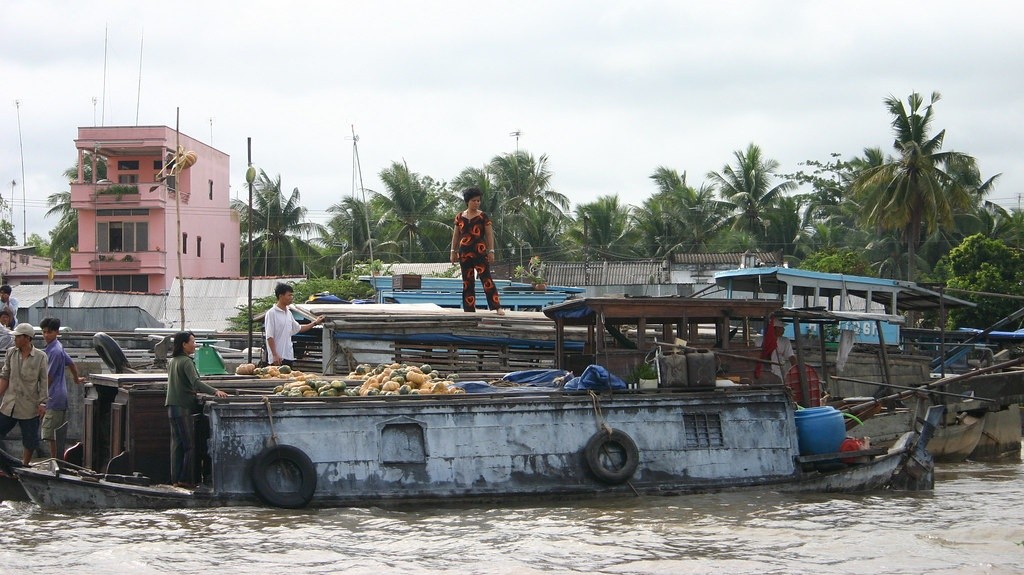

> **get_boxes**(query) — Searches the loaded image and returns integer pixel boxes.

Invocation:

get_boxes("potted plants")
[96,186,141,200]
[371,260,384,276]
[513,254,548,291]
[638,363,658,390]
[88,254,142,269]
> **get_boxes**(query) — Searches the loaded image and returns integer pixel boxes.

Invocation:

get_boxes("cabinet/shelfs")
[392,274,421,292]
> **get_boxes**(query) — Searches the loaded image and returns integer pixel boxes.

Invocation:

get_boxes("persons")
[265,284,323,371]
[450,187,506,315]
[755,320,796,377]
[0,323,50,465]
[39,318,86,458]
[0,282,18,328]
[164,331,228,487]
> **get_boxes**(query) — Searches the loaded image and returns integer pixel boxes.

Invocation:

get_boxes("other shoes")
[173,481,198,488]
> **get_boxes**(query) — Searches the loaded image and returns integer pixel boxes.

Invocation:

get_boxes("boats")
[1,264,1024,513]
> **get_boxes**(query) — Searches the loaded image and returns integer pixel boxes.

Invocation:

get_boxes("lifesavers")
[91,329,132,374]
[250,443,317,510]
[582,428,641,486]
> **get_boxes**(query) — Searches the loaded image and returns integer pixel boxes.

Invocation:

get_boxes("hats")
[775,318,788,327]
[8,322,35,338]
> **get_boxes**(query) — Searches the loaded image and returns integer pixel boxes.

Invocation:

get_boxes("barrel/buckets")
[659,352,688,386]
[687,348,716,386]
[794,406,847,456]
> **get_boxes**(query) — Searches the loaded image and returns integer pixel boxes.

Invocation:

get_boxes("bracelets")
[314,322,316,326]
[489,250,494,253]
[451,250,456,252]
